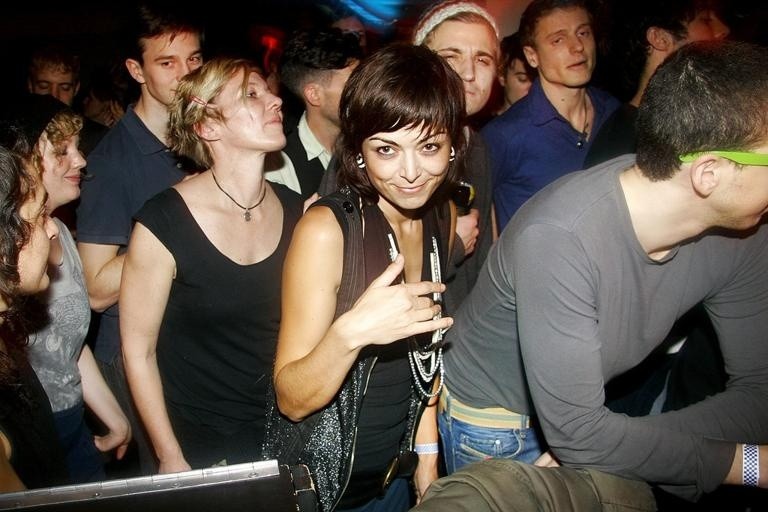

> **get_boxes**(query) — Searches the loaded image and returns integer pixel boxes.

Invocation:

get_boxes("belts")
[441,383,537,429]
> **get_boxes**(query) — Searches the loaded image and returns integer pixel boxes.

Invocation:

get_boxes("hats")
[0,92,68,156]
[412,0,500,46]
